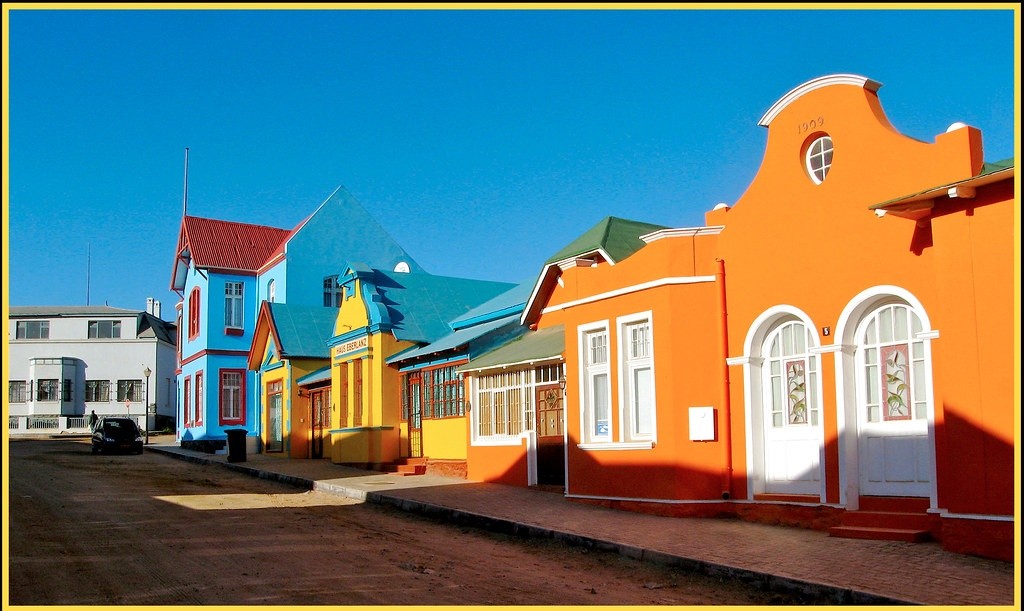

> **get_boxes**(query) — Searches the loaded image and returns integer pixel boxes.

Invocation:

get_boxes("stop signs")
[126,399,130,407]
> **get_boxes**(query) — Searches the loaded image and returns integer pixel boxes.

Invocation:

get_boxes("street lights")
[144,367,151,444]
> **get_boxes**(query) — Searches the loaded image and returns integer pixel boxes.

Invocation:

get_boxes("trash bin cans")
[224,429,248,463]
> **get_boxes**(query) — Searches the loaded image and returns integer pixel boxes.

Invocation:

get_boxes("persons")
[89,410,98,432]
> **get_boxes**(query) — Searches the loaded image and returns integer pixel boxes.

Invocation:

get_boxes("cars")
[92,416,144,454]
